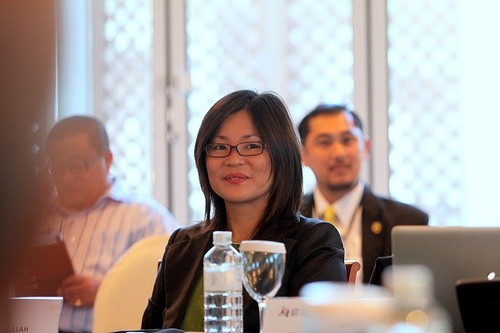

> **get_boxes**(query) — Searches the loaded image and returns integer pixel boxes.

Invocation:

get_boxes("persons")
[141,90,348,333]
[298,103,428,285]
[6,116,181,333]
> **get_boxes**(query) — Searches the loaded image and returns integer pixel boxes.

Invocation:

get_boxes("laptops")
[391,226,500,333]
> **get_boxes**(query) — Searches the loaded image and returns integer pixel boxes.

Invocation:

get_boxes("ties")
[323,206,335,224]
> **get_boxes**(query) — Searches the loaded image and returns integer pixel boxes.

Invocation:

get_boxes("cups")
[456,279,500,333]
[298,280,396,333]
[5,296,64,333]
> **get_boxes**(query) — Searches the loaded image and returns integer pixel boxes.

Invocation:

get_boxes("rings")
[75,299,82,307]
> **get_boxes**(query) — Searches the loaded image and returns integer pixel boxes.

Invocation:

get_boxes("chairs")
[93,234,171,333]
[391,225,500,333]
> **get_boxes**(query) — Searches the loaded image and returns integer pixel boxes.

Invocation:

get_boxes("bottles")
[377,263,456,333]
[202,231,244,333]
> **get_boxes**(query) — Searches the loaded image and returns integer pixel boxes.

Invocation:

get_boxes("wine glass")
[239,240,286,333]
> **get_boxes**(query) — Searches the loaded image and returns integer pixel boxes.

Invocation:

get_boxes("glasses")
[48,150,106,176]
[205,142,266,158]
